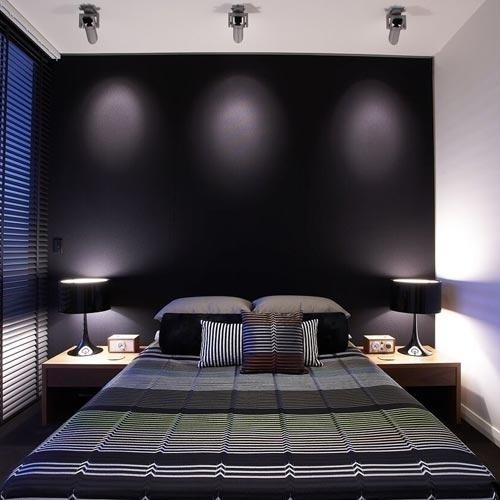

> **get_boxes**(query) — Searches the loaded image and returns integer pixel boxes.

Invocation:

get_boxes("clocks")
[107,334,140,353]
[363,334,396,354]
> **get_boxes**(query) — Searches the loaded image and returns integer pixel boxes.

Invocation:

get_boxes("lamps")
[78,4,102,44]
[57,277,111,356]
[389,277,442,356]
[383,6,406,46]
[228,4,248,43]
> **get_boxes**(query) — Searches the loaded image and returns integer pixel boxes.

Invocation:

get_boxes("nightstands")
[42,346,151,425]
[355,345,461,424]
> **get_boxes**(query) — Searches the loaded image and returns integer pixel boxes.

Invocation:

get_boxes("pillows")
[152,294,352,375]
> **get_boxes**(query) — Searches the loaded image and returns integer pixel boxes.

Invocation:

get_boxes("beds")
[0,340,500,500]
